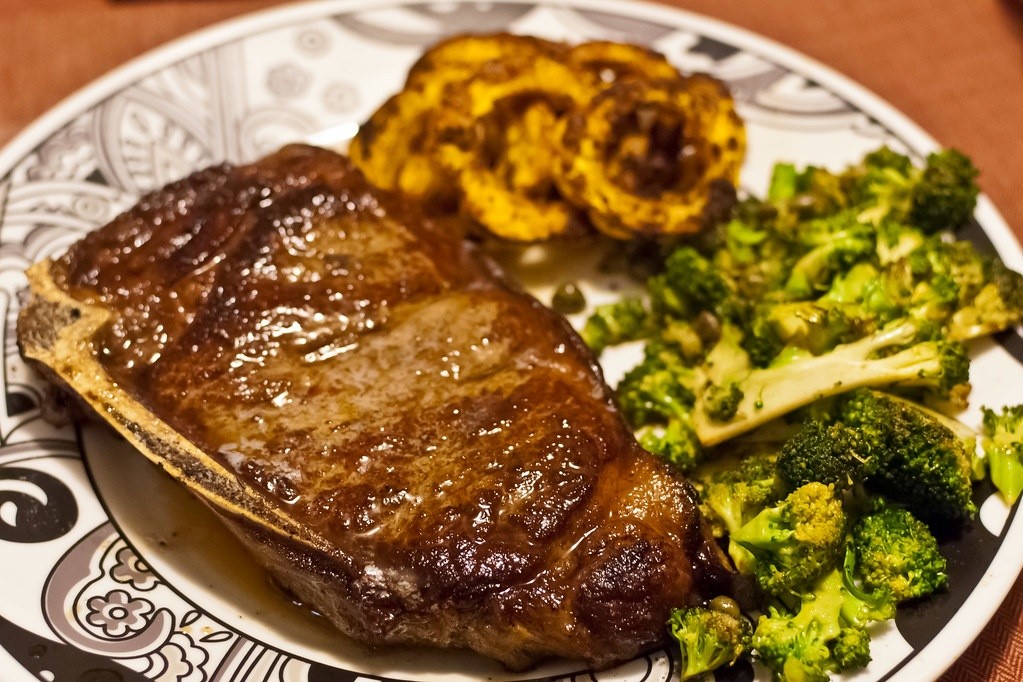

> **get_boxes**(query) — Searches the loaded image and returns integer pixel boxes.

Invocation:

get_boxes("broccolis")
[579,146,1023,682]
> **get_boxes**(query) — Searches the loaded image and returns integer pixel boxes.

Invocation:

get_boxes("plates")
[0,0,1023,682]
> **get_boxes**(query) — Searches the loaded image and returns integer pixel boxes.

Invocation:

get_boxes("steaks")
[11,144,733,674]
